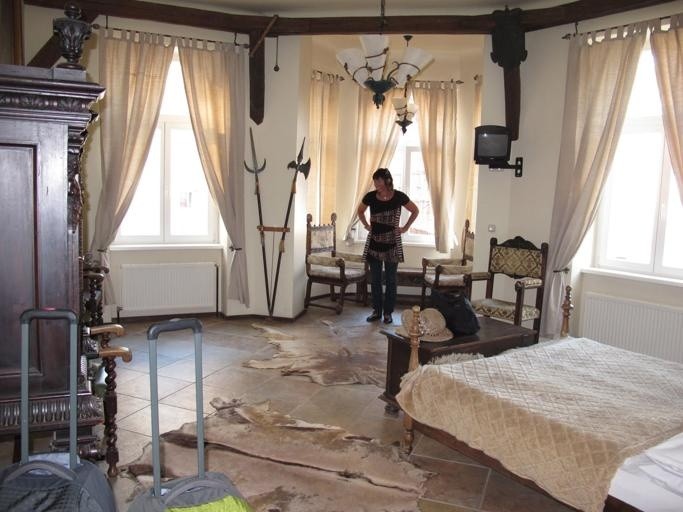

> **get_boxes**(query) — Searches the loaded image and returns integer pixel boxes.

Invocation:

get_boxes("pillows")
[637,459,683,495]
[643,429,682,475]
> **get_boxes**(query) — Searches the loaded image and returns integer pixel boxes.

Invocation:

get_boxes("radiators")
[119,262,222,318]
[581,290,683,366]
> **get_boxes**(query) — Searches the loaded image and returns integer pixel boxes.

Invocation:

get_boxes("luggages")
[125,317,257,512]
[0,306,118,512]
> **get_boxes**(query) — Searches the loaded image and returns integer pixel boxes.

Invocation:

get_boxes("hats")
[399,307,454,343]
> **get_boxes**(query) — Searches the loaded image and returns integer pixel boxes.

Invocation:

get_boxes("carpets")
[119,397,437,511]
[245,312,460,387]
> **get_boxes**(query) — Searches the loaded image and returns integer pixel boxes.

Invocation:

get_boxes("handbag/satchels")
[420,290,481,337]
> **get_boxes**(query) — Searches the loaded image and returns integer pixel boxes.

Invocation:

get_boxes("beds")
[390,280,683,512]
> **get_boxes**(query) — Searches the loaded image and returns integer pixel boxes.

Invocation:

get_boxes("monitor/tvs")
[474,125,512,164]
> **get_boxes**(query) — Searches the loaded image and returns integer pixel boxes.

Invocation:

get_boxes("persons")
[356,167,418,323]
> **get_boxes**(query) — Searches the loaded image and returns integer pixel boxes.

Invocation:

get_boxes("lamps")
[334,0,434,106]
[392,92,419,133]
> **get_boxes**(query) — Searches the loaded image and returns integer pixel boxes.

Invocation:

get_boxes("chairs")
[420,218,480,305]
[459,236,549,344]
[304,213,374,315]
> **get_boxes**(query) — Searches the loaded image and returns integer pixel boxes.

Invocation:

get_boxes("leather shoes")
[382,312,392,324]
[365,311,382,323]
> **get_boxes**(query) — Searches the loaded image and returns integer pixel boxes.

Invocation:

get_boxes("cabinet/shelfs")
[1,64,131,478]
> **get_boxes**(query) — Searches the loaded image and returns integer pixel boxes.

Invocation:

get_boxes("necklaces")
[379,192,391,201]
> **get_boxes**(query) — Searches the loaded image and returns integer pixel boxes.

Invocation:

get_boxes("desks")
[365,265,422,306]
[379,318,542,412]
